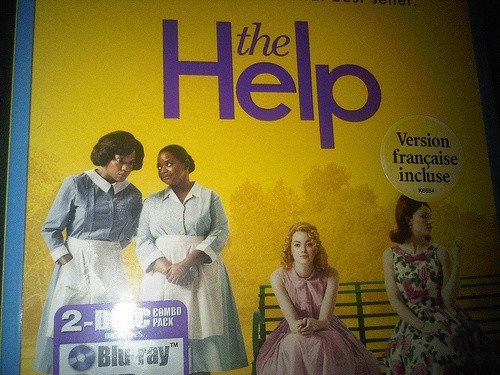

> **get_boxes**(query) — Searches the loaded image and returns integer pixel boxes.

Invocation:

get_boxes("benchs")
[251,274,500,375]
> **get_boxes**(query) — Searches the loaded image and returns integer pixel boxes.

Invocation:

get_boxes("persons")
[135,145,249,375]
[256,222,381,375]
[34,131,144,373]
[381,195,500,375]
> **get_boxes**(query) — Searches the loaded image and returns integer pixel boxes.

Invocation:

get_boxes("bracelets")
[417,320,427,331]
